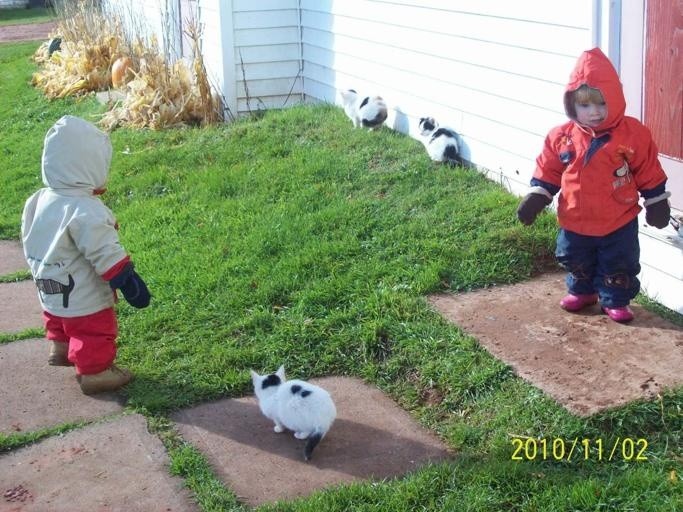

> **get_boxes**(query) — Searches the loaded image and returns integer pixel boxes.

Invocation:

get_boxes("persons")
[20,114,150,394]
[518,47,671,324]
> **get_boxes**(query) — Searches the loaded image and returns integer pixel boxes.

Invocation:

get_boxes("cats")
[341,88,388,133]
[418,116,462,168]
[250,363,337,464]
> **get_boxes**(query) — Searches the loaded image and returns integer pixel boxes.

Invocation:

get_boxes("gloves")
[516,185,552,225]
[110,260,151,308]
[643,191,671,230]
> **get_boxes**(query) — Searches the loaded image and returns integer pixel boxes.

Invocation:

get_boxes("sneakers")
[80,365,131,395]
[601,301,634,323]
[47,339,75,366]
[559,292,598,312]
